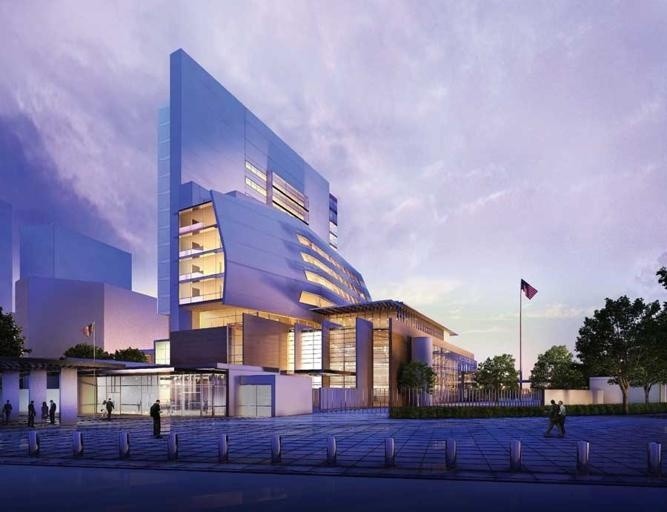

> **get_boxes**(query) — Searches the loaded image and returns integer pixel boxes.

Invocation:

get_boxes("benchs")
[79,323,93,337]
[520,277,537,300]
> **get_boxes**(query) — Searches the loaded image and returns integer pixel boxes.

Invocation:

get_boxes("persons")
[203,397,208,413]
[2,400,12,424]
[99,399,109,419]
[105,398,114,420]
[543,399,565,438]
[39,401,48,426]
[27,400,36,427]
[49,400,56,424]
[149,399,162,436]
[558,400,566,433]
